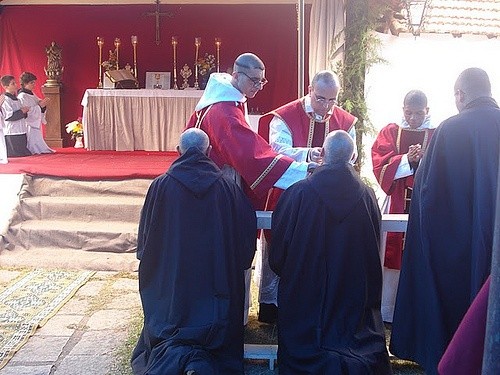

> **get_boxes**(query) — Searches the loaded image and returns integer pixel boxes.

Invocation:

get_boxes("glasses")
[311,89,338,105]
[233,72,268,87]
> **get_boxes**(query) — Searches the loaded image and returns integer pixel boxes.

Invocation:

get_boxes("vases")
[74,137,83,148]
[199,76,209,90]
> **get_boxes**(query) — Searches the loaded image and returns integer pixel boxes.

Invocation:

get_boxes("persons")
[267,130,395,375]
[0,71,56,165]
[181,54,444,329]
[129,128,257,375]
[388,69,500,375]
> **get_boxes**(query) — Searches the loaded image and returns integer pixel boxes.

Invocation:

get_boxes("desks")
[243,210,408,370]
[80,89,205,152]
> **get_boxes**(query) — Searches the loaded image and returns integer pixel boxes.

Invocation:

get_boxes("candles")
[171,36,178,44]
[96,36,105,46]
[114,37,121,47]
[214,36,222,45]
[131,35,138,43]
[194,37,201,47]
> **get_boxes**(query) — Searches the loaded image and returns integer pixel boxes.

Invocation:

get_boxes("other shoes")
[384,322,392,330]
[258,302,279,323]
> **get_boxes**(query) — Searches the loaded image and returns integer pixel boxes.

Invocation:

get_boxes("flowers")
[102,48,118,71]
[193,52,216,76]
[65,120,84,140]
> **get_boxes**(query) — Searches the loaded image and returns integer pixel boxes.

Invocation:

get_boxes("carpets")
[0,269,97,370]
